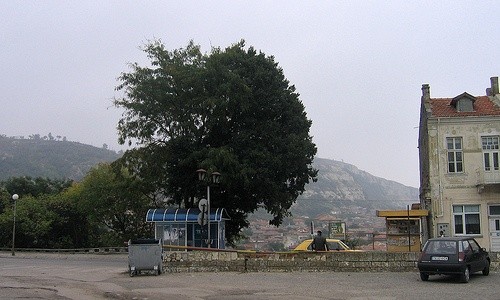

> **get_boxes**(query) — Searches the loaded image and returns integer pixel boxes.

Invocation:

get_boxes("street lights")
[196,168,223,248]
[11,194,19,255]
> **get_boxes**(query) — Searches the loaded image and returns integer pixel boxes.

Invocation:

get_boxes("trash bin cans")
[127,239,161,276]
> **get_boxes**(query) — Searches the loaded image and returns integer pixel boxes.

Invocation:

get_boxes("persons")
[312,231,326,251]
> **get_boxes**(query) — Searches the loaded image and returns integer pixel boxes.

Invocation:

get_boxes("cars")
[417,236,491,283]
[294,238,351,251]
